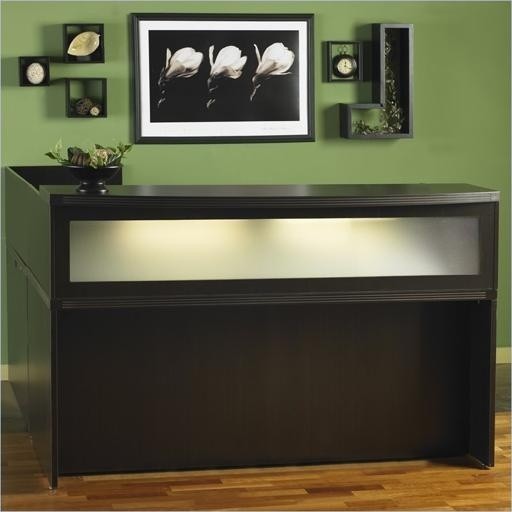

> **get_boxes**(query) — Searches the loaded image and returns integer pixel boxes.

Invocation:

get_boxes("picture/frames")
[129,14,316,143]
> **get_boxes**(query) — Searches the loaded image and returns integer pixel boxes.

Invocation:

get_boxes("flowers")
[46,140,133,167]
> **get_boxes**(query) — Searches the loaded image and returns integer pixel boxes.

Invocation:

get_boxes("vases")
[68,165,122,193]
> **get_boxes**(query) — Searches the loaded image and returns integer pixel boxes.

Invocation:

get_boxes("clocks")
[323,40,364,83]
